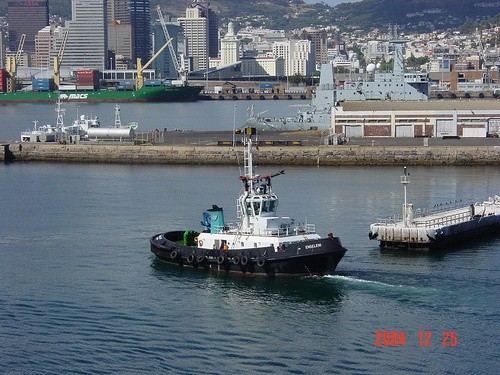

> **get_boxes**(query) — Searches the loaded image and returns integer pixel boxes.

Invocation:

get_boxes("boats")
[368,166,500,253]
[150,103,347,277]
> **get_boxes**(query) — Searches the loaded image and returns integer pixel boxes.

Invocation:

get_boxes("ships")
[0,5,204,104]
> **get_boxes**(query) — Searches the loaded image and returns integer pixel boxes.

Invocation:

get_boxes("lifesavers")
[199,240,203,246]
